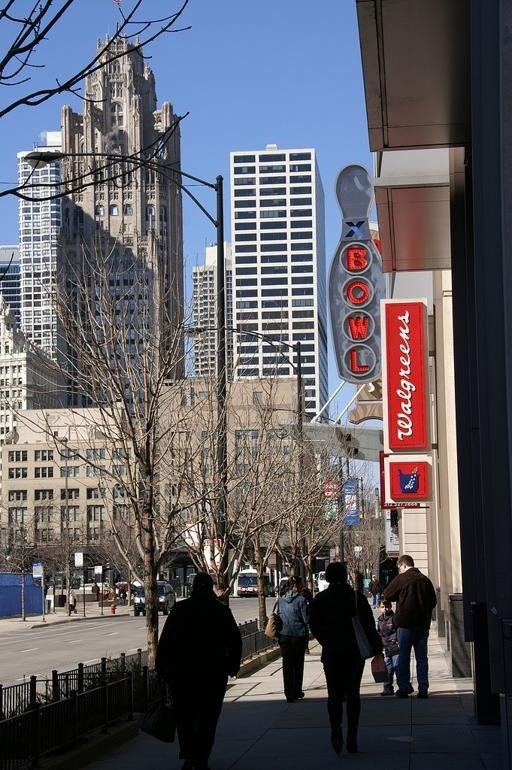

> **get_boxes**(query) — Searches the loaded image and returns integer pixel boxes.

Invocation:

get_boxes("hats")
[372,575,376,578]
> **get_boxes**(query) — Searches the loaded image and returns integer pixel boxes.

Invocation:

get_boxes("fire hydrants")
[110,603,117,614]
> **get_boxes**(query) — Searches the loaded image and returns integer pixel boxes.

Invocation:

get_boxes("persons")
[275,575,312,705]
[368,573,382,610]
[152,570,246,767]
[304,562,385,756]
[381,553,445,698]
[66,571,182,613]
[375,596,401,696]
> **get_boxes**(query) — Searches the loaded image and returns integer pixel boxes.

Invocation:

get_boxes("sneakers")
[380,691,394,696]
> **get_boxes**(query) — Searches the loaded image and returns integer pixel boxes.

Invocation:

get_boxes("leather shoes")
[395,683,415,697]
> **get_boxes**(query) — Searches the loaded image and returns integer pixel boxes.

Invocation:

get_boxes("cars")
[44,582,131,595]
[275,570,330,597]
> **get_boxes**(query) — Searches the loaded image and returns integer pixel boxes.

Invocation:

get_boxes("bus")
[236,569,270,598]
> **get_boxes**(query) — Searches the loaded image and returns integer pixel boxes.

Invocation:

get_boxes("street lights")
[184,324,306,584]
[262,405,346,562]
[24,150,232,622]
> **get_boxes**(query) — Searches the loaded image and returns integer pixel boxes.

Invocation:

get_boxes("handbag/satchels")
[264,601,281,639]
[370,652,388,682]
[138,685,182,744]
[352,590,375,660]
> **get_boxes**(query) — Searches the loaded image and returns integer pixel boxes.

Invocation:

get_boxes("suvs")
[132,578,177,617]
